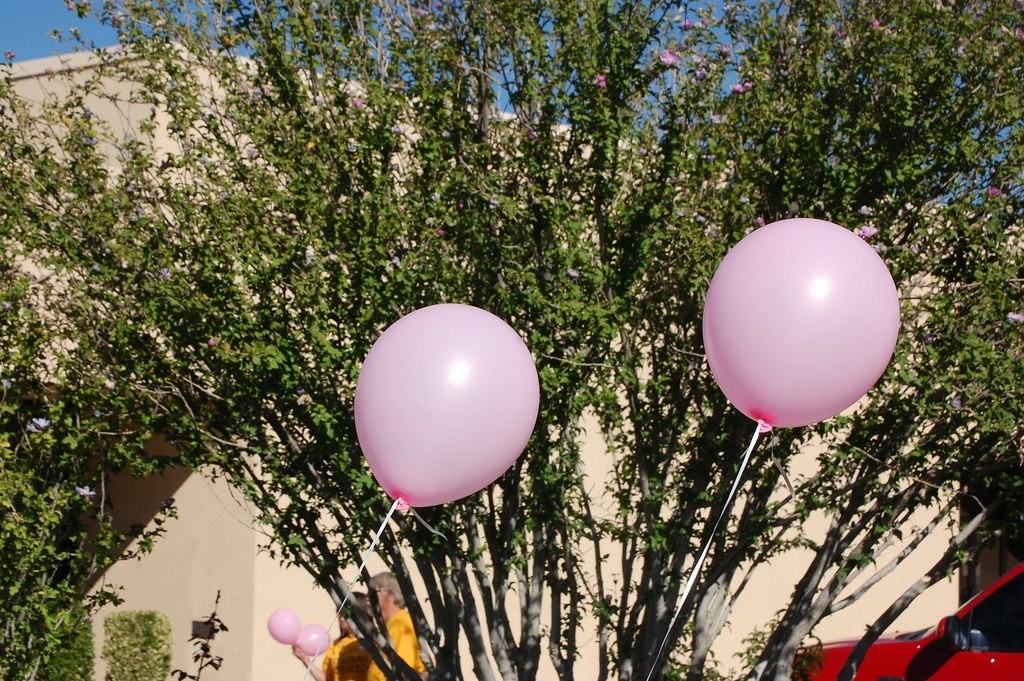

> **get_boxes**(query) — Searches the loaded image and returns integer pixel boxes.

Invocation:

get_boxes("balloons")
[354,303,540,510]
[267,607,300,644]
[295,623,330,656]
[702,218,900,433]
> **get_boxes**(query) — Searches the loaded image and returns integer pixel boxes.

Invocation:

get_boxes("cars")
[793,561,1024,681]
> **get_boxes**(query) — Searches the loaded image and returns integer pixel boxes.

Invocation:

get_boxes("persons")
[367,572,427,681]
[292,591,372,681]
